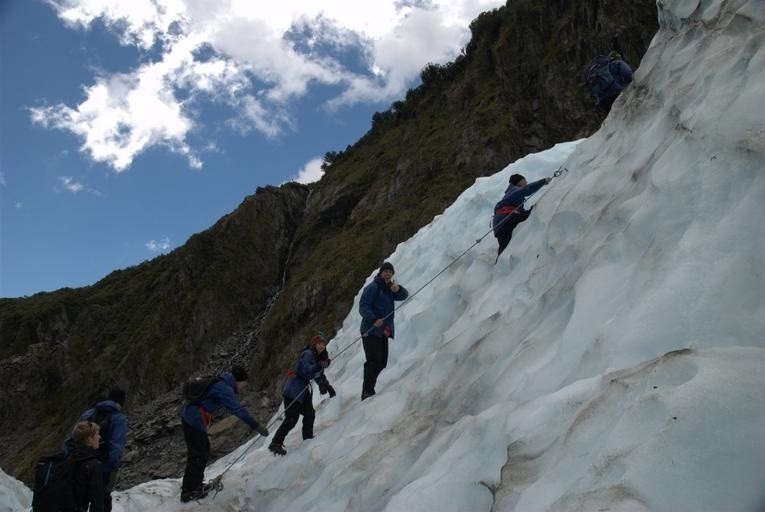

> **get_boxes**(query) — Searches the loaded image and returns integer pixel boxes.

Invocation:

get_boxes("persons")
[601,49,636,116]
[74,385,128,511]
[177,362,269,503]
[492,173,552,264]
[29,419,105,511]
[266,334,337,456]
[357,261,410,401]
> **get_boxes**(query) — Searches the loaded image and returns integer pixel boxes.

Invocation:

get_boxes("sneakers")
[179,483,210,502]
[268,442,287,455]
[361,392,376,400]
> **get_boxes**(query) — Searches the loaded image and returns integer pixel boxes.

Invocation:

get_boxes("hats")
[508,174,524,185]
[312,335,326,346]
[379,261,395,276]
[108,387,125,402]
[232,365,249,382]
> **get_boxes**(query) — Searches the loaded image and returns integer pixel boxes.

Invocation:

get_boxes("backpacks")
[87,407,118,440]
[182,375,222,404]
[31,454,96,512]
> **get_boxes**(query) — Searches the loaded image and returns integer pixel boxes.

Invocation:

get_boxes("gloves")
[320,358,330,368]
[255,423,268,437]
[319,384,336,397]
